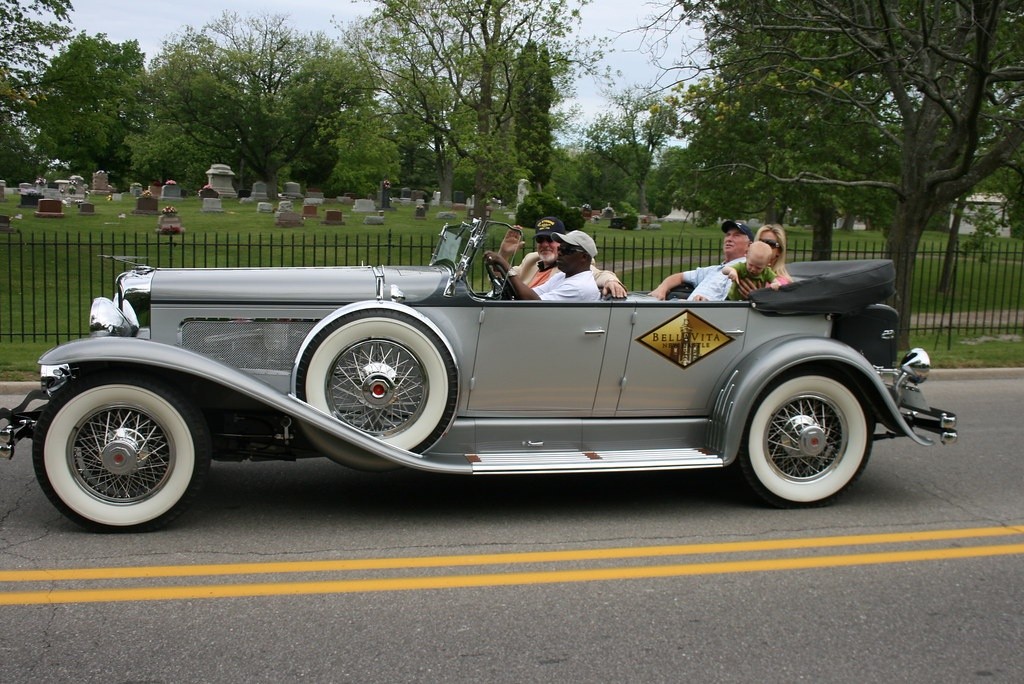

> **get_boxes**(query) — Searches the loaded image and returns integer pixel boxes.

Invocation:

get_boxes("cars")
[0,215,961,540]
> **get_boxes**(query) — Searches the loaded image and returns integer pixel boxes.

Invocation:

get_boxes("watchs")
[507,269,517,278]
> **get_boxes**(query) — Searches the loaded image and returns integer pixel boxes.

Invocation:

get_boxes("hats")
[552,230,598,258]
[722,221,753,242]
[532,217,566,239]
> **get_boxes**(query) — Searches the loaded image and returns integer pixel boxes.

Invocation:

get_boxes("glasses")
[759,240,781,249]
[535,238,554,243]
[556,246,585,255]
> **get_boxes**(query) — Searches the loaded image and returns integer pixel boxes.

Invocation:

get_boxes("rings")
[489,257,492,260]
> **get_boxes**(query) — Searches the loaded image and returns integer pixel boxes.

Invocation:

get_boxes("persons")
[489,217,628,300]
[693,224,792,302]
[722,242,780,301]
[647,221,754,302]
[483,230,601,300]
